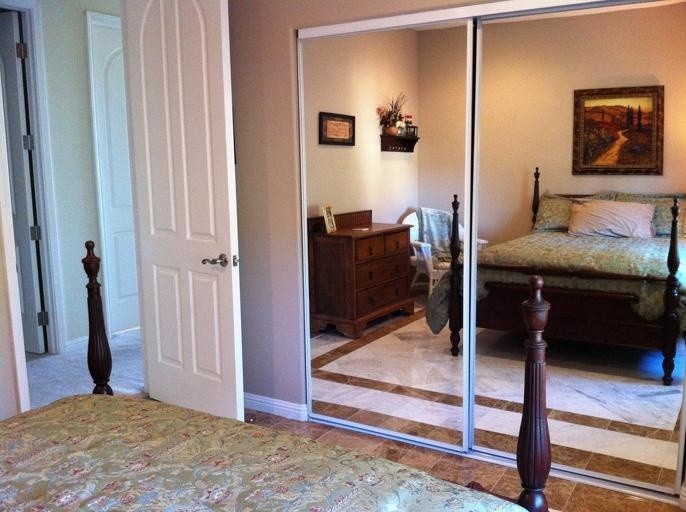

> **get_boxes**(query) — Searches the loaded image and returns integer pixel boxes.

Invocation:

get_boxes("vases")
[384,126,398,135]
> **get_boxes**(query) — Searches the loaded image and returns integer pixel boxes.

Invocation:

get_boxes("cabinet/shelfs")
[309,222,415,339]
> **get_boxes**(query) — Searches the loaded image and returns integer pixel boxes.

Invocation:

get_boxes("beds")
[0,391,558,511]
[449,192,686,385]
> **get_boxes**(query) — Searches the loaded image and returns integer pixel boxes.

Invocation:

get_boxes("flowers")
[377,92,406,127]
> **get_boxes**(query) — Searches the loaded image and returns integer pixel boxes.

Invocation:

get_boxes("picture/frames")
[318,112,356,147]
[572,88,666,174]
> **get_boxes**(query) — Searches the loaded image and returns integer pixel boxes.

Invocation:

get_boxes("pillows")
[536,192,681,239]
